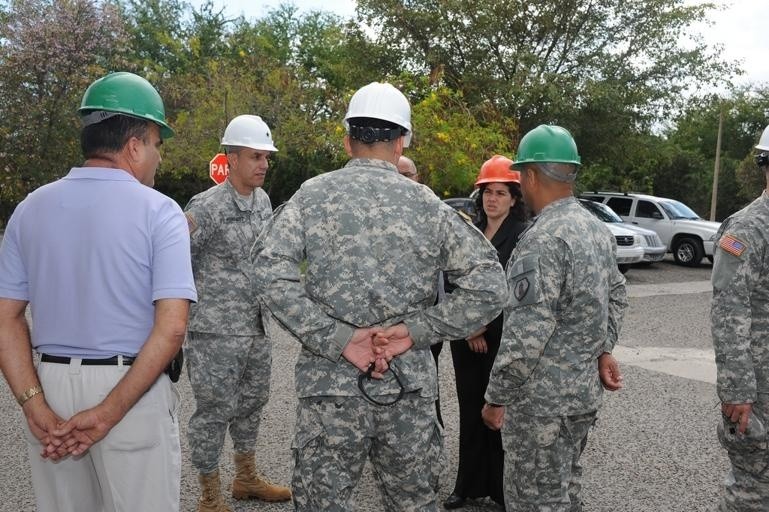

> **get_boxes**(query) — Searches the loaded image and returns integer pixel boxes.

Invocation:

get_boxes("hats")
[717,409,764,455]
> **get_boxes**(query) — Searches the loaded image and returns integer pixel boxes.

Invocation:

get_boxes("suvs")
[581,190,724,267]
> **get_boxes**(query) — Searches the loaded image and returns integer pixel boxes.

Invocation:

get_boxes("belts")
[40,353,138,366]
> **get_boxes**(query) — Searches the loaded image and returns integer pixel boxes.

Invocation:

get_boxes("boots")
[231,448,292,503]
[195,467,235,512]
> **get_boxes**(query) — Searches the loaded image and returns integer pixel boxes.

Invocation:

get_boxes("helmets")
[509,124,581,171]
[473,153,520,186]
[219,114,280,153]
[341,79,413,149]
[754,123,769,152]
[76,70,177,139]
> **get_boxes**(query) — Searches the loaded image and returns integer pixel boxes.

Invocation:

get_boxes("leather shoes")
[444,490,467,509]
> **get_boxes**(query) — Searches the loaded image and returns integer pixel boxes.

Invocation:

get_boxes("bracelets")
[12,385,44,405]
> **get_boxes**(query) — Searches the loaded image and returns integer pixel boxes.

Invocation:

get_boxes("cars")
[583,200,666,264]
[441,197,476,215]
[575,198,643,274]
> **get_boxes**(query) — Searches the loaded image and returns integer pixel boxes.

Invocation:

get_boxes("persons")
[179,114,291,512]
[249,79,507,512]
[1,72,199,512]
[482,124,628,512]
[441,155,532,510]
[393,156,465,432]
[711,118,769,510]
